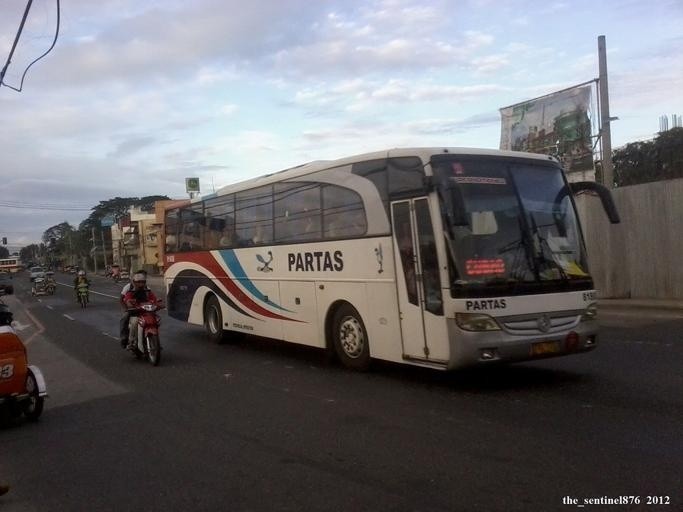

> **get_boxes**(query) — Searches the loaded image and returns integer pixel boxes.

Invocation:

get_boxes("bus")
[0,257,22,273]
[161,146,623,377]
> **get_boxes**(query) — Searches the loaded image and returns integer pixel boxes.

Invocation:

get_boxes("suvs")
[29,266,45,282]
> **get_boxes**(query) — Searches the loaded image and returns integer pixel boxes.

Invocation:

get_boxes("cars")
[63,265,86,276]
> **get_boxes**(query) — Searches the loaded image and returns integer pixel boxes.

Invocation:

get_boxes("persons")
[164,204,552,308]
[73,270,87,285]
[124,274,156,349]
[118,270,146,350]
[46,275,53,282]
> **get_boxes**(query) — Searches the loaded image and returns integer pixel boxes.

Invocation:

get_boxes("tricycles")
[0,296,49,419]
[113,273,120,283]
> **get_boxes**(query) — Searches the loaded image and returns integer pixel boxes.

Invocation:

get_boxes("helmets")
[132,273,146,290]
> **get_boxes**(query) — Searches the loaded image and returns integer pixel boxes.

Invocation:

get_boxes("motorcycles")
[73,279,92,311]
[47,280,57,295]
[120,299,166,365]
